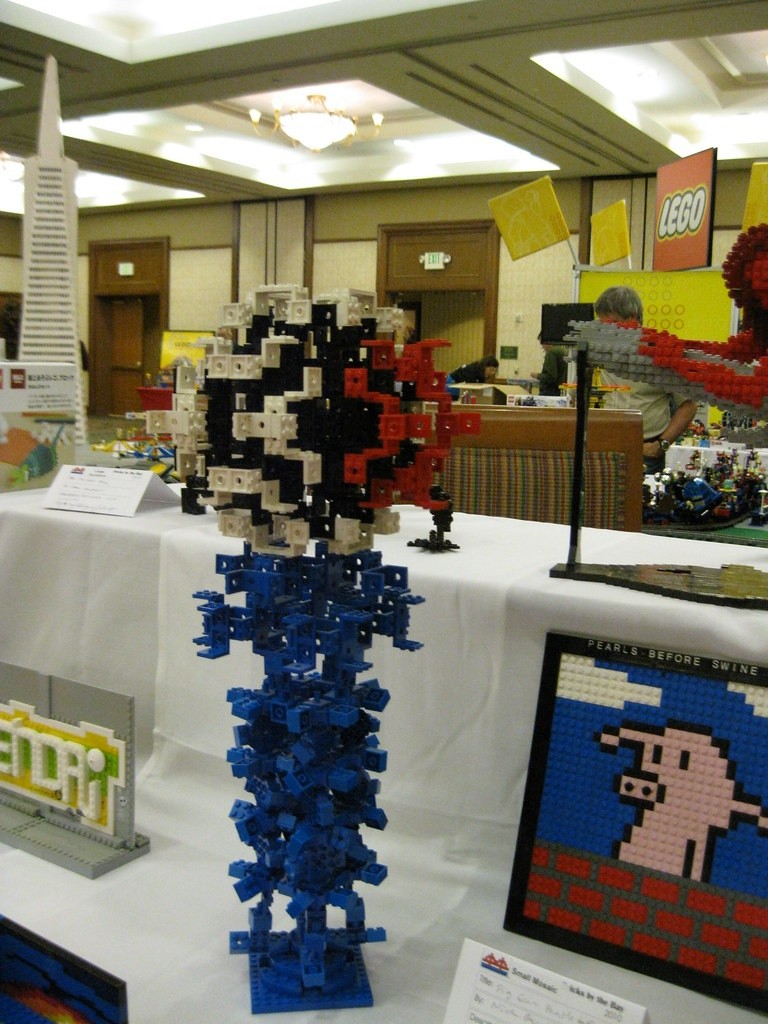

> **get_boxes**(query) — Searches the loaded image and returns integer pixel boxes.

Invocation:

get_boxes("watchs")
[658,438,670,452]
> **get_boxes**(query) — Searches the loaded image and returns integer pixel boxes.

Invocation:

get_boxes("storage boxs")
[0,415,77,495]
[0,360,78,415]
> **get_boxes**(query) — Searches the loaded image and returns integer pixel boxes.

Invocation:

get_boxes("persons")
[591,286,695,475]
[445,356,500,401]
[530,331,568,396]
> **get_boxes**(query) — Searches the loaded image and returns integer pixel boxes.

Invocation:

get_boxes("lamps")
[251,91,383,154]
